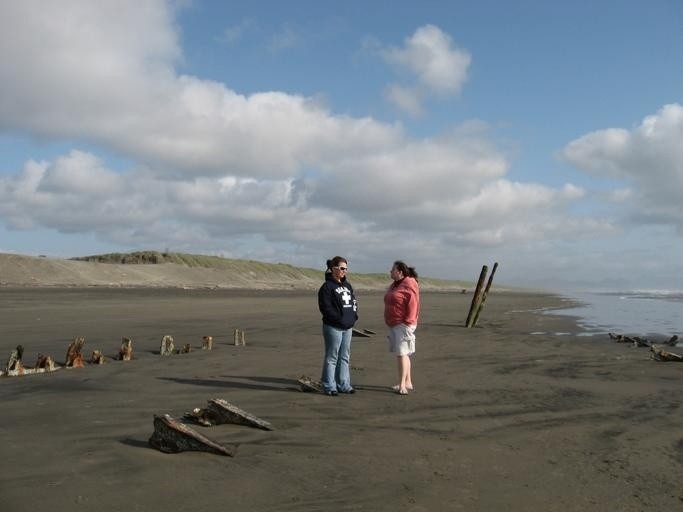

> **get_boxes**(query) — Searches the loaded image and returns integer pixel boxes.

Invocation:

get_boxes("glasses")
[333,266,347,271]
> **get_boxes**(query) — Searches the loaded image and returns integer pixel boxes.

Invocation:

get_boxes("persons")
[317,256,358,396]
[384,262,419,396]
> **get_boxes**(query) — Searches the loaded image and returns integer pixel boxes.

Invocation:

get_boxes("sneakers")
[392,384,412,394]
[324,384,338,395]
[340,384,355,394]
